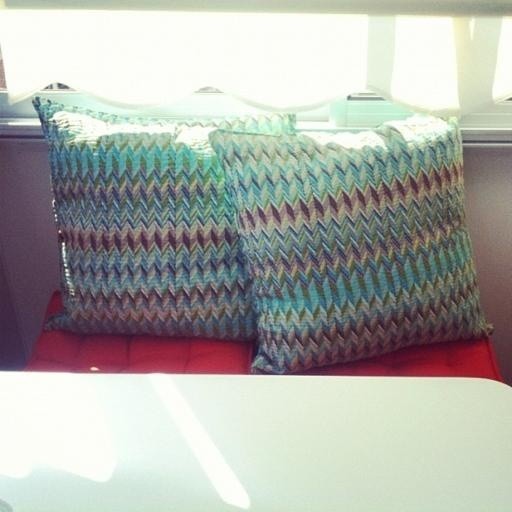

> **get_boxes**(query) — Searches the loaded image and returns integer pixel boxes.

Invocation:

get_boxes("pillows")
[204,115,492,374]
[29,94,299,342]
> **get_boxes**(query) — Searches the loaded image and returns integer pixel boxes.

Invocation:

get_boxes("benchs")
[24,289,503,382]
